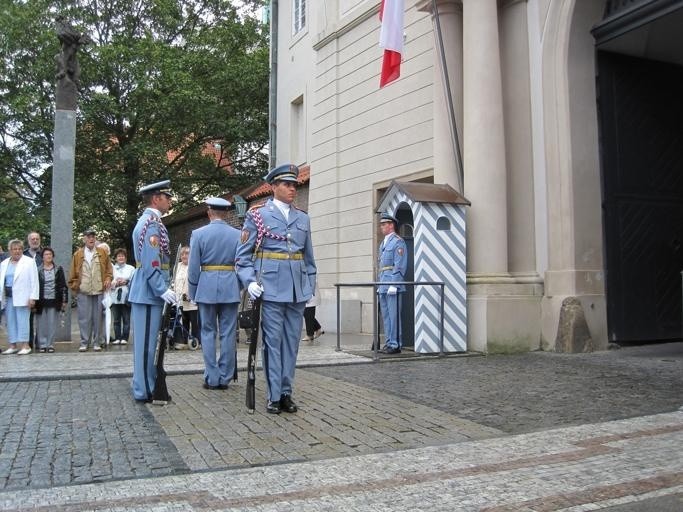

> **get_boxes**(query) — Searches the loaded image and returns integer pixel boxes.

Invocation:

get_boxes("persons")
[113,248,135,344]
[234,163,317,414]
[0,245,9,323]
[128,180,176,403]
[377,212,408,354]
[173,247,198,339]
[23,232,43,351]
[37,248,68,353]
[94,243,111,346]
[302,291,324,341]
[0,240,40,355]
[188,197,243,390]
[69,229,114,351]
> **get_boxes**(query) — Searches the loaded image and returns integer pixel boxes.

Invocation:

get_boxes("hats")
[265,164,300,185]
[205,196,231,211]
[139,179,173,198]
[83,229,97,237]
[380,211,399,226]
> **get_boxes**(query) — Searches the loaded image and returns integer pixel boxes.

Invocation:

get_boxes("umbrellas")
[102,285,112,346]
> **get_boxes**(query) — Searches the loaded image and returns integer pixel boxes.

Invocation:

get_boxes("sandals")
[39,347,46,353]
[48,347,55,353]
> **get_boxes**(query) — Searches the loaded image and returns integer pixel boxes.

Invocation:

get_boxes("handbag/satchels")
[238,289,254,328]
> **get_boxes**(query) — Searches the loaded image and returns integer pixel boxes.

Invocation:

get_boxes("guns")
[152,281,170,405]
[246,271,262,413]
[372,291,380,350]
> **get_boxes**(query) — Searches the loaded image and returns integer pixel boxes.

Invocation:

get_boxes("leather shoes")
[378,344,389,353]
[281,394,298,412]
[382,347,401,354]
[266,399,282,414]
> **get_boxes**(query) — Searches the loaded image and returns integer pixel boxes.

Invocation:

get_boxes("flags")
[379,1,406,89]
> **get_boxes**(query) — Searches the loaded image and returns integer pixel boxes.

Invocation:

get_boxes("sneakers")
[301,335,315,341]
[17,346,32,355]
[78,346,90,351]
[112,339,121,345]
[120,339,128,345]
[2,347,18,355]
[93,345,101,351]
[314,327,325,340]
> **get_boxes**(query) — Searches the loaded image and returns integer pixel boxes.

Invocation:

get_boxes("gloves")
[247,281,265,300]
[387,285,399,296]
[160,289,178,305]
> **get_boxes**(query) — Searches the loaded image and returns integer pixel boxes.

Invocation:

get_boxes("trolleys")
[166,305,199,353]
